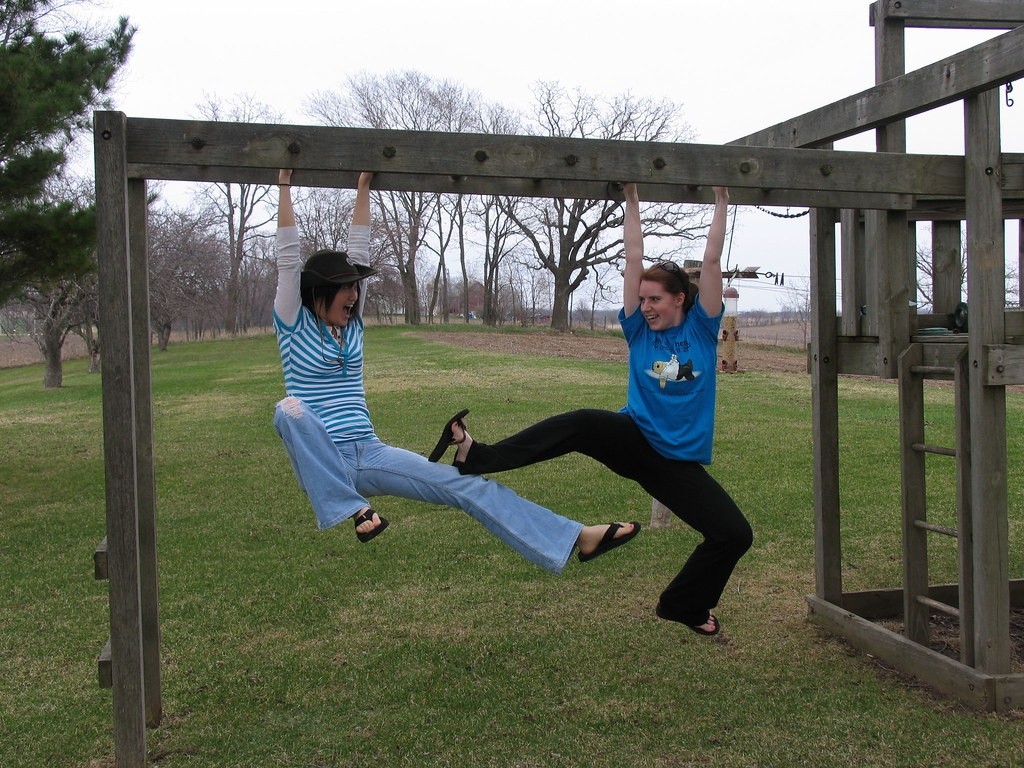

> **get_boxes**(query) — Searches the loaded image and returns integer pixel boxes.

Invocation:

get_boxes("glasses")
[656,261,684,292]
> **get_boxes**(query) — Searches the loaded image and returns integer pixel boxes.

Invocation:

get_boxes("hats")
[300,249,378,291]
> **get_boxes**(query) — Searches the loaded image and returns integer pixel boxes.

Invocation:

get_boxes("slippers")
[355,509,389,544]
[577,521,641,563]
[428,408,470,464]
[655,596,720,635]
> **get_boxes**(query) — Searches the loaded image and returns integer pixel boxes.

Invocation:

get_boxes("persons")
[428,182,754,636]
[271,168,641,575]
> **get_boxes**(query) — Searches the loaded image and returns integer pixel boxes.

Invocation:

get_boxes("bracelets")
[277,184,292,189]
